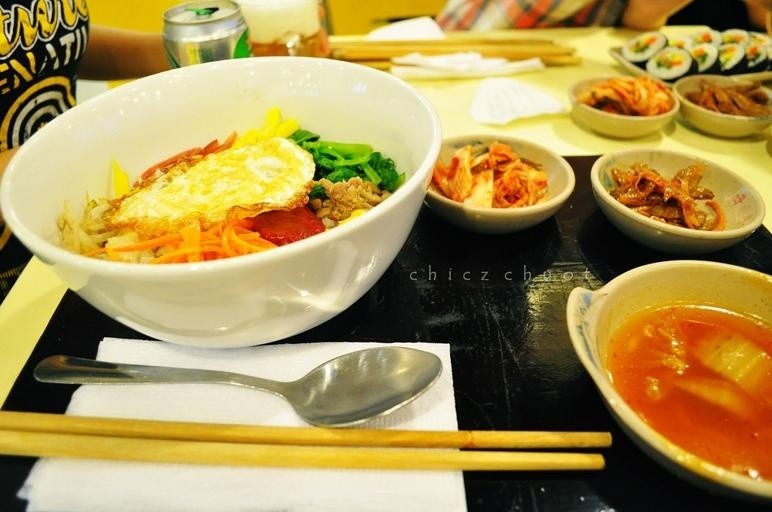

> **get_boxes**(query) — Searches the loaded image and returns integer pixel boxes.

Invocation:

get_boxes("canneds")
[162,0,252,71]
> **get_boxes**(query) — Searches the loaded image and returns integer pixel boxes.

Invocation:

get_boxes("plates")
[605,45,772,89]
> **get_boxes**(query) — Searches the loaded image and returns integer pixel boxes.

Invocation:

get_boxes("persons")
[434,0,772,33]
[0,0,331,307]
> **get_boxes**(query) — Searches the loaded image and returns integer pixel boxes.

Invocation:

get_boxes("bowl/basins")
[564,259,772,508]
[425,127,578,237]
[672,74,772,139]
[588,145,766,258]
[566,73,681,141]
[0,55,443,347]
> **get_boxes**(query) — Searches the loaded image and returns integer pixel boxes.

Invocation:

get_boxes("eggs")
[100,134,317,239]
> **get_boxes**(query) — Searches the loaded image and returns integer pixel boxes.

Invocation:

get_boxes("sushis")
[622,27,772,82]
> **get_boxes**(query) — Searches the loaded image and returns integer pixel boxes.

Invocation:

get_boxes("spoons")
[33,344,444,429]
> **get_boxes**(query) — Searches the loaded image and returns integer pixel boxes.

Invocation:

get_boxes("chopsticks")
[326,37,584,67]
[0,408,620,470]
